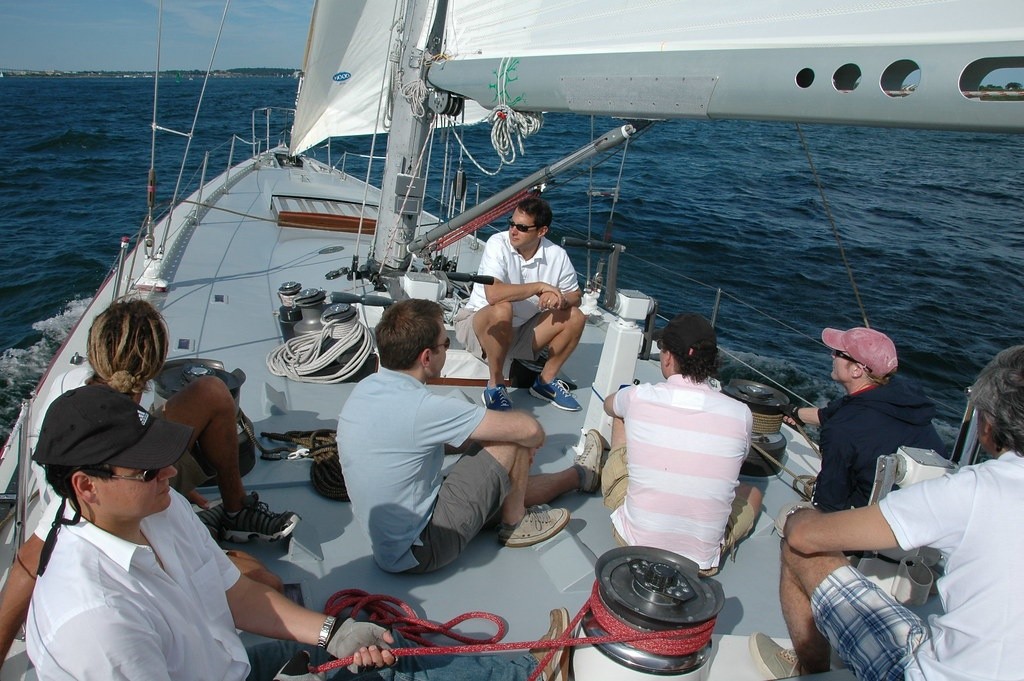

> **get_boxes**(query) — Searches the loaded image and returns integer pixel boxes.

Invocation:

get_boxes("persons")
[456,197,586,410]
[748,345,1024,681]
[335,300,603,575]
[25,387,570,681]
[0,299,298,670]
[603,313,762,576]
[775,327,948,511]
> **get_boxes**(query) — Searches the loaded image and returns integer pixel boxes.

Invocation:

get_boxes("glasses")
[93,469,159,482]
[833,350,872,373]
[507,216,536,232]
[656,339,665,350]
[430,337,450,349]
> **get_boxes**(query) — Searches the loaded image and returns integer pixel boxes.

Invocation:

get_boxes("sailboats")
[0,0,1024,670]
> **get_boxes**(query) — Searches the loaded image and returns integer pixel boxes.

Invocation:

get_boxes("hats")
[822,327,898,379]
[31,384,195,469]
[651,312,717,356]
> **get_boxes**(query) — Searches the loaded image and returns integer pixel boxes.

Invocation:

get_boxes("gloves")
[775,502,815,538]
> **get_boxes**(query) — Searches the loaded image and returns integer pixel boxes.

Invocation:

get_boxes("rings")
[548,300,550,302]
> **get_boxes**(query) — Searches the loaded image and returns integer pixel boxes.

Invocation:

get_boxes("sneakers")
[482,381,513,410]
[497,507,569,546]
[749,633,800,680]
[575,429,603,493]
[222,491,302,543]
[529,374,583,411]
[529,606,570,681]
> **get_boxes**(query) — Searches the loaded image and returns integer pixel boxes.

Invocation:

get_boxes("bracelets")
[318,616,336,648]
